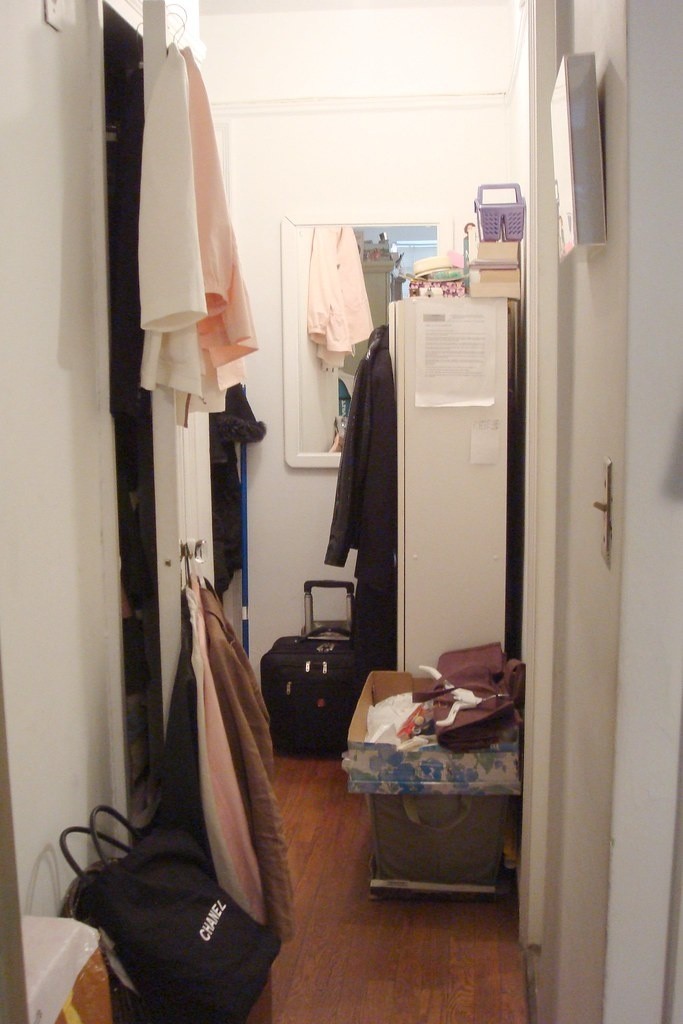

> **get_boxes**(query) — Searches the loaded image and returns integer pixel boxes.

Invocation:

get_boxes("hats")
[412,255,453,279]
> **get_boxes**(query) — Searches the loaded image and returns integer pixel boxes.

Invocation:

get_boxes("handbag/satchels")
[60,804,283,1024]
[412,640,526,755]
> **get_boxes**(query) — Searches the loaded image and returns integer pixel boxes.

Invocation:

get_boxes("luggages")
[300,578,355,640]
[260,624,357,752]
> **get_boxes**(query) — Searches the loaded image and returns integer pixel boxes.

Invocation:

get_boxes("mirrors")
[279,213,455,468]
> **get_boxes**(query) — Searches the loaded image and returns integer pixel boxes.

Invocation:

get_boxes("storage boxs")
[340,670,523,898]
[472,182,526,243]
[467,242,522,301]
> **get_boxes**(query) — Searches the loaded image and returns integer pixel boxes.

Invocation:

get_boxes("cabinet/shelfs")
[339,259,404,375]
[386,296,509,678]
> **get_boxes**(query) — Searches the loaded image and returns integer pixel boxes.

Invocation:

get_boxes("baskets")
[474,183,525,243]
